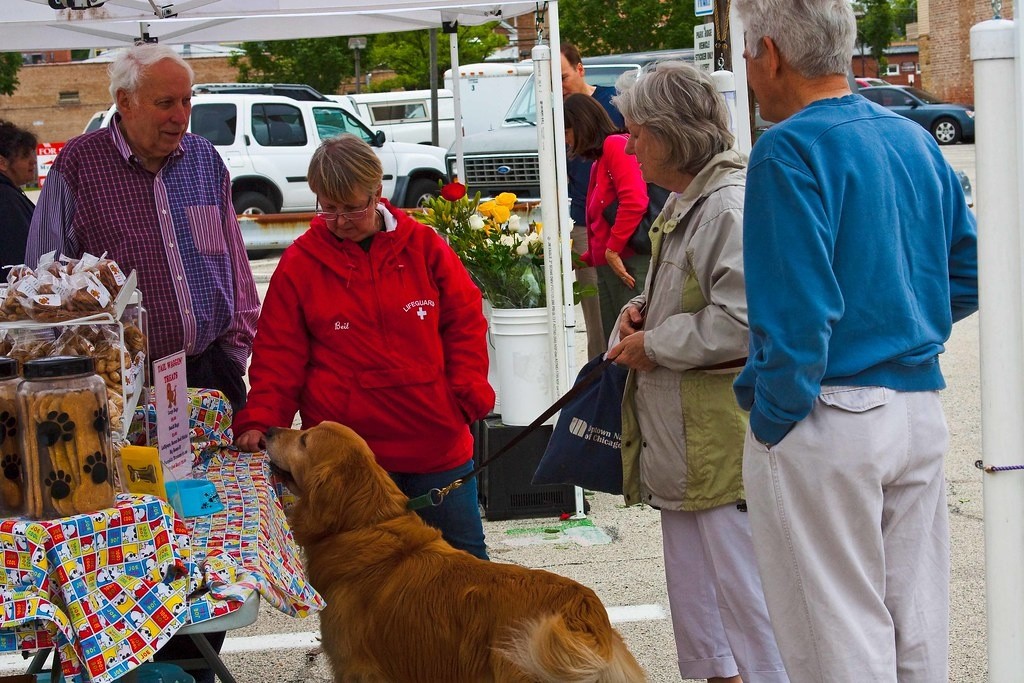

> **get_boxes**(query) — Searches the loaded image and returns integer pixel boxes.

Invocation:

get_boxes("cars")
[858,84,975,146]
[855,76,890,95]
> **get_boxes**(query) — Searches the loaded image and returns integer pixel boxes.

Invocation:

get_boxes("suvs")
[75,80,455,260]
[448,45,702,206]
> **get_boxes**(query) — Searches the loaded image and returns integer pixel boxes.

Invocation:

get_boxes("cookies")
[0,259,147,433]
[0,381,114,518]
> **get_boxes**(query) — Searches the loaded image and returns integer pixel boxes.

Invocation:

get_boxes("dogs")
[261,421,649,683]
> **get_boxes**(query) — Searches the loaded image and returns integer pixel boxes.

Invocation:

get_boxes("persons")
[551,42,630,363]
[0,118,38,284]
[606,62,790,682]
[563,92,652,348]
[26,43,261,682]
[234,134,495,561]
[734,0,980,683]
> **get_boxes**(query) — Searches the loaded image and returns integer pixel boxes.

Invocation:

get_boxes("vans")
[333,56,548,151]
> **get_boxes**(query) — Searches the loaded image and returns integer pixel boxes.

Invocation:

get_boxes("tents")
[0,0,584,519]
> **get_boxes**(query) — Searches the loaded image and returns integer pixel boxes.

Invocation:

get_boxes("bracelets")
[607,248,617,254]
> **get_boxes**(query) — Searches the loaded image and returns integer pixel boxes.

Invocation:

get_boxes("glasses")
[315,191,373,220]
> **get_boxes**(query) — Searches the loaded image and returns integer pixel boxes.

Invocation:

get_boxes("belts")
[187,344,215,376]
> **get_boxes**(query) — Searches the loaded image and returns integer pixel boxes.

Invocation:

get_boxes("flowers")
[405,173,596,308]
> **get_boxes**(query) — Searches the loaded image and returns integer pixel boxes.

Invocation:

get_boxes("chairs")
[271,122,292,145]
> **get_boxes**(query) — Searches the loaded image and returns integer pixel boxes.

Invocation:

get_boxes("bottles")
[119,287,150,405]
[0,355,29,517]
[15,354,116,521]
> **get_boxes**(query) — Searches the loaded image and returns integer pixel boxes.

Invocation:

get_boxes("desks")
[0,454,328,683]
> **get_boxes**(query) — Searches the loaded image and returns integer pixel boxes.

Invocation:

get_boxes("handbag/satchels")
[602,135,673,255]
[531,303,640,496]
